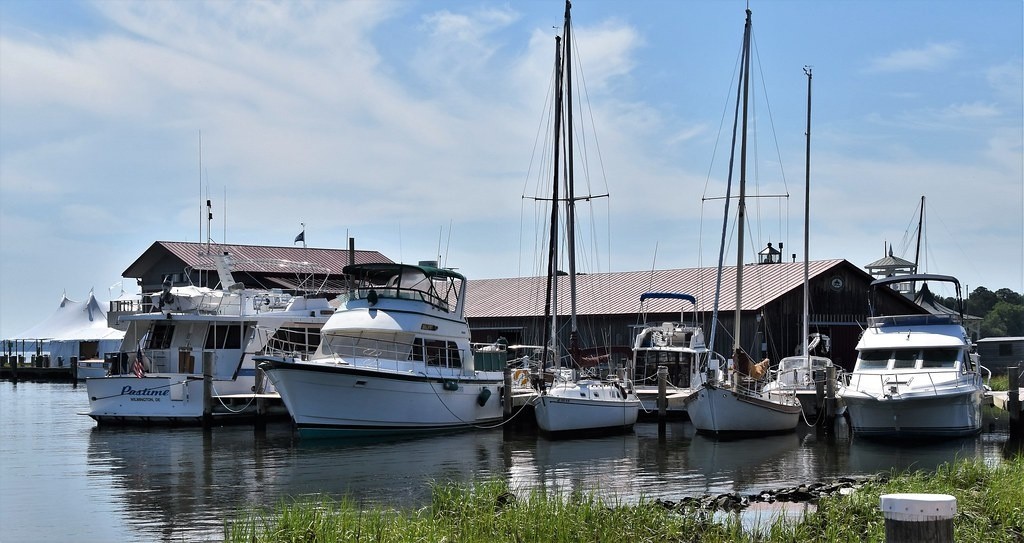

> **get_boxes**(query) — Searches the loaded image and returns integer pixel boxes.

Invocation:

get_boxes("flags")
[295,230,305,243]
[134,344,145,378]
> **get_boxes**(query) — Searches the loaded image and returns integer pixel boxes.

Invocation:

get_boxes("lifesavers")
[514,370,530,383]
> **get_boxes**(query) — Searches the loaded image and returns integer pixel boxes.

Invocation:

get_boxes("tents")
[1,285,143,367]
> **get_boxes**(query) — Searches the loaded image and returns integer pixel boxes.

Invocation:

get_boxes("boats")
[839,274,984,436]
[980,387,1024,429]
[76,283,337,422]
[509,363,577,411]
[625,294,705,413]
[253,260,508,430]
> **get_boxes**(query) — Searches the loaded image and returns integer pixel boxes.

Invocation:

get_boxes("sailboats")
[533,1,642,433]
[763,64,850,415]
[682,7,803,430]
